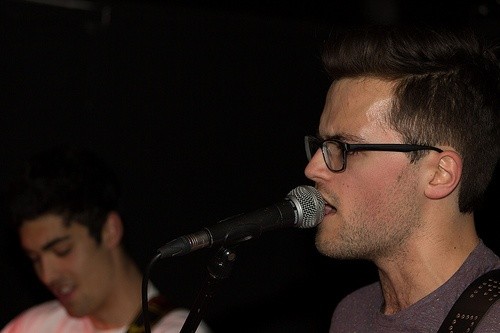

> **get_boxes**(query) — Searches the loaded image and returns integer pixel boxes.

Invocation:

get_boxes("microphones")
[159,186,325,258]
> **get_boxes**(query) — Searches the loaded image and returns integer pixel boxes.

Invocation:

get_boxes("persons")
[305,26,500,333]
[0,179,209,333]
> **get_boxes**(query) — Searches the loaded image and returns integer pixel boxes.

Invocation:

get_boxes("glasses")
[303,135,444,173]
